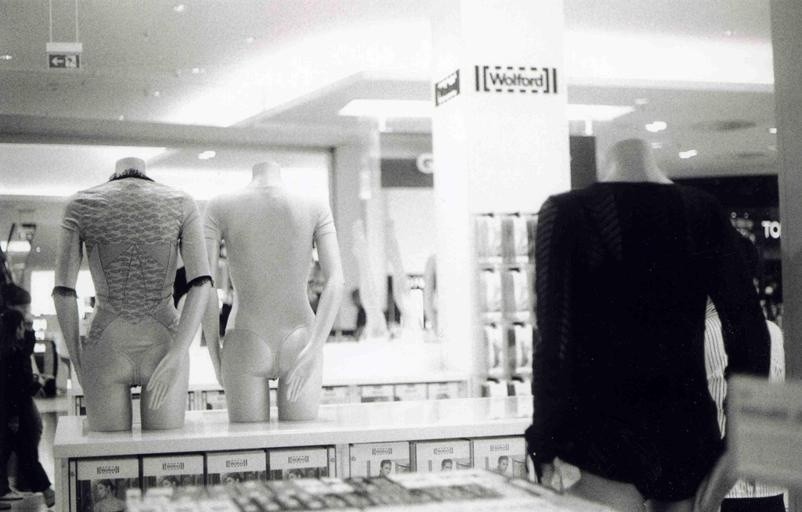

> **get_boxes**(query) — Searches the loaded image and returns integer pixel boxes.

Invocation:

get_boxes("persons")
[527,136,771,511]
[0,282,54,507]
[203,156,346,422]
[52,156,215,433]
